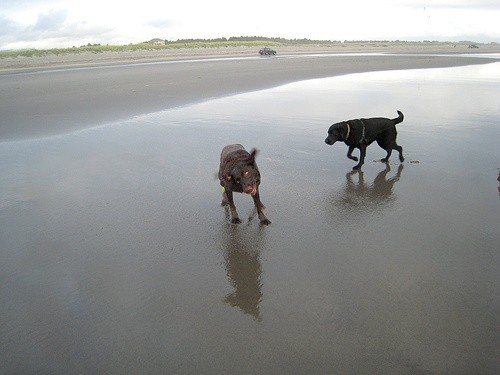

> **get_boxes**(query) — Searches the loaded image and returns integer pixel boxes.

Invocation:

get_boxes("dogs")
[217,143,272,226]
[324,109,404,171]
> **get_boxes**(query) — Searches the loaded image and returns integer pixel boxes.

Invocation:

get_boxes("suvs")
[259,48,276,56]
